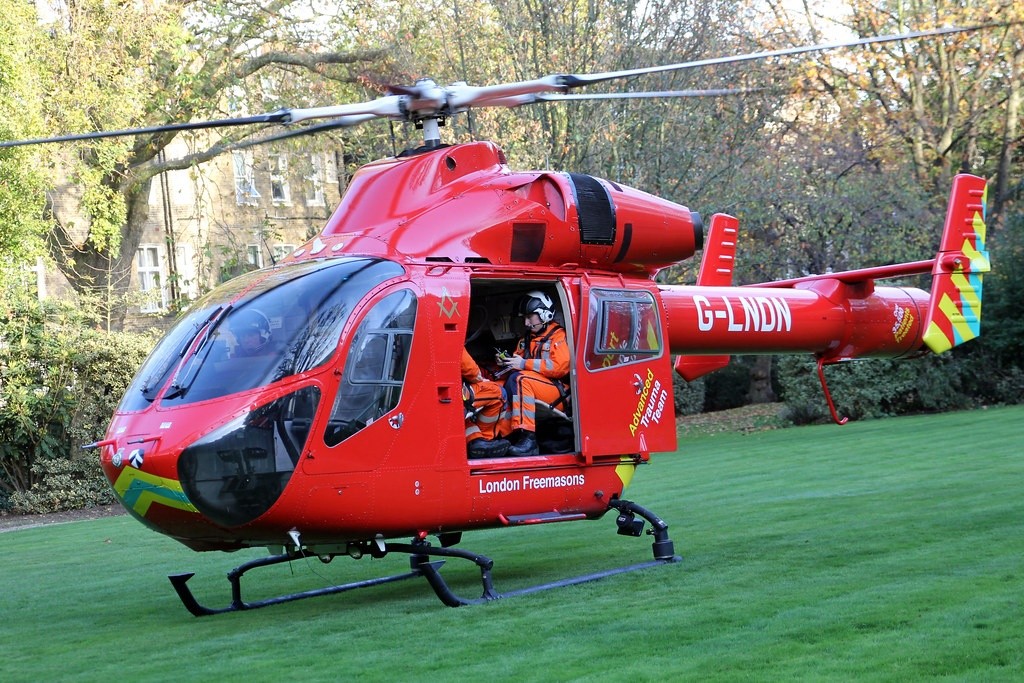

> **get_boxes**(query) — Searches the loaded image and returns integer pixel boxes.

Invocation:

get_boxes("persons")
[496,291,572,456]
[229,309,274,358]
[460,346,511,458]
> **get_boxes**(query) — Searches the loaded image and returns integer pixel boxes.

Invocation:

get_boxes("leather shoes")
[509,438,539,456]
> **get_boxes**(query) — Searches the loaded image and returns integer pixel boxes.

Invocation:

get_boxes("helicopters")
[0,22,1024,617]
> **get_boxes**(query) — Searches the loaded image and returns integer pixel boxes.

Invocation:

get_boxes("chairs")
[533,398,573,423]
[355,338,388,379]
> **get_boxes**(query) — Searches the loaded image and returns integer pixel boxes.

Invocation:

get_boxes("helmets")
[517,290,556,324]
[229,309,273,345]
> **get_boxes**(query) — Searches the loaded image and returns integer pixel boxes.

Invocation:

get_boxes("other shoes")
[470,439,510,457]
